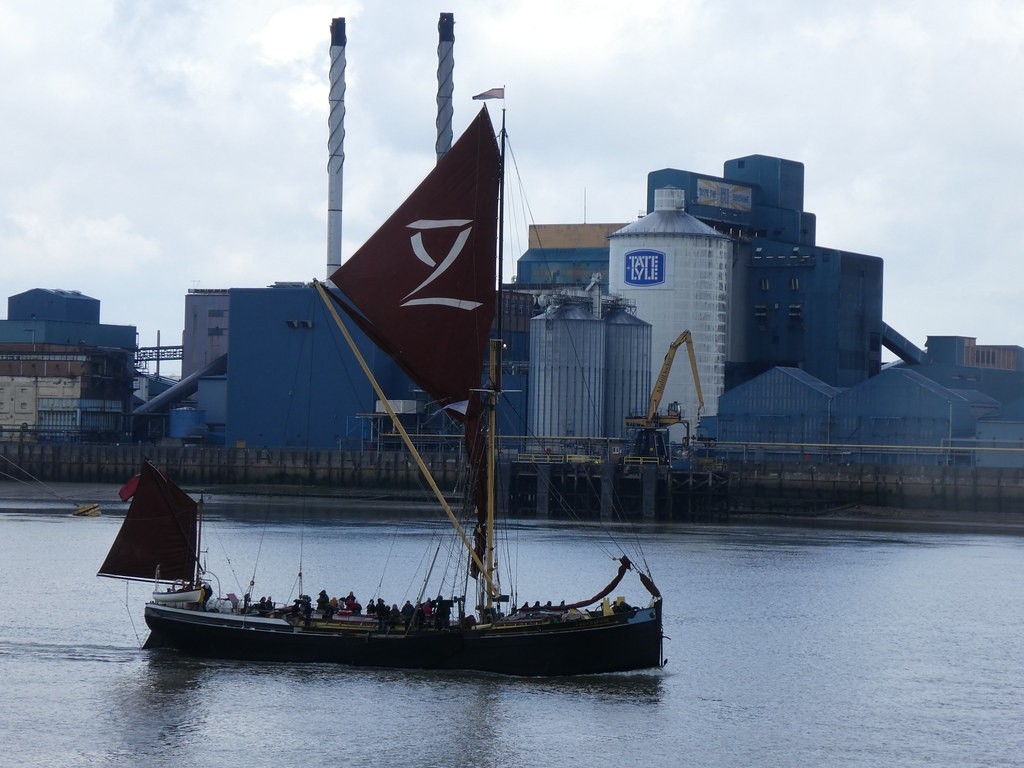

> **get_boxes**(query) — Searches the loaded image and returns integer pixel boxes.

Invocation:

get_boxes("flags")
[472,88,504,100]
[95,458,196,586]
[333,101,501,580]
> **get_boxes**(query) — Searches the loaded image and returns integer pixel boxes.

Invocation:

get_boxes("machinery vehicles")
[622,329,706,465]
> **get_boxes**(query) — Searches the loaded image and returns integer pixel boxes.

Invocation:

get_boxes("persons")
[156,577,636,629]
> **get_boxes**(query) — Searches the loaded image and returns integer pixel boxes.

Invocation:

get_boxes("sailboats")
[96,86,668,677]
